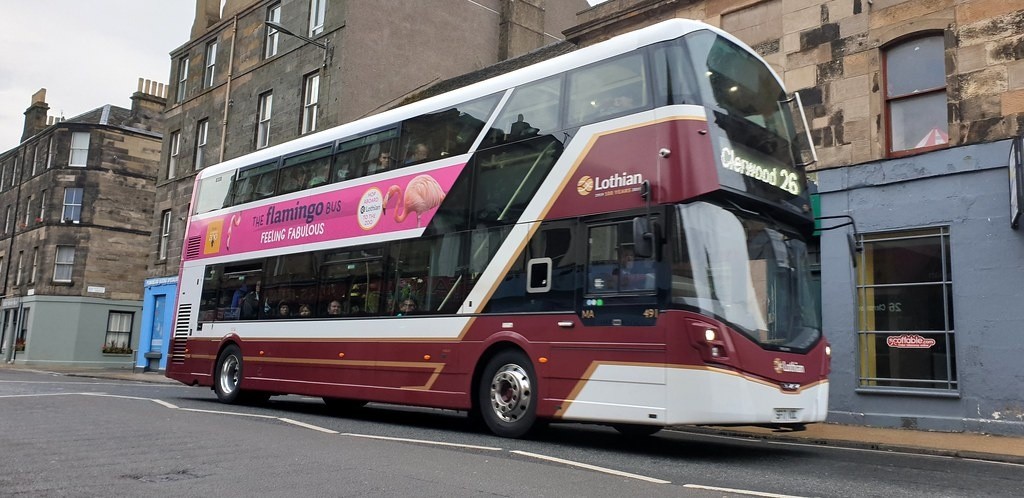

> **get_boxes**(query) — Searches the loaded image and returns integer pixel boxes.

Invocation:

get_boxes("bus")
[163,17,864,437]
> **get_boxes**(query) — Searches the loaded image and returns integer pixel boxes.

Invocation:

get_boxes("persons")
[377,150,389,170]
[611,248,654,289]
[337,159,349,178]
[275,299,291,318]
[232,276,278,320]
[299,304,312,316]
[399,295,418,311]
[327,300,343,314]
[406,144,430,163]
[308,163,329,185]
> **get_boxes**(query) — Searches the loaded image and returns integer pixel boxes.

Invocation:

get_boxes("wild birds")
[382,175,446,228]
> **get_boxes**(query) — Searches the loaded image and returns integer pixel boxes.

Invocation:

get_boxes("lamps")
[265,21,334,64]
